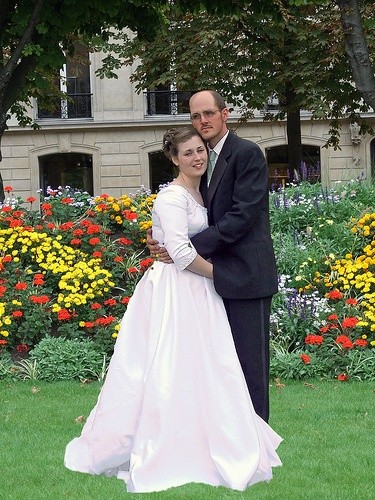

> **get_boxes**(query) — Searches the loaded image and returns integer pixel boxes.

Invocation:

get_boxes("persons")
[62,123,288,495]
[146,90,280,423]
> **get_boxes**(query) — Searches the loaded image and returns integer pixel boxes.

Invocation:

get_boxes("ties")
[207,151,216,186]
